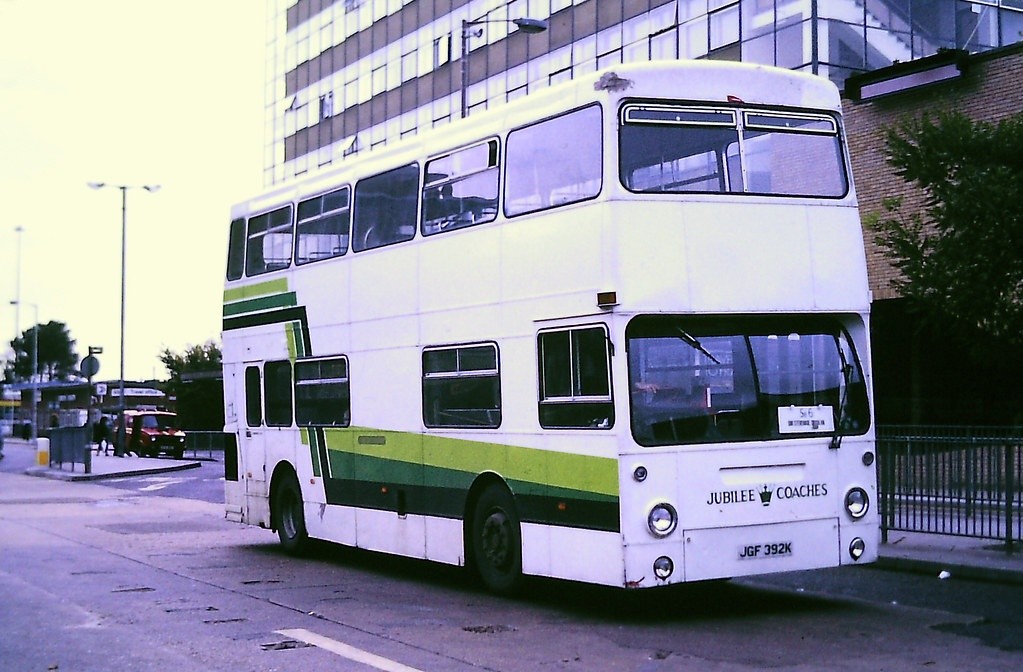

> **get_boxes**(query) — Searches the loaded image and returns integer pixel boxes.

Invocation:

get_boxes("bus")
[222,60,878,595]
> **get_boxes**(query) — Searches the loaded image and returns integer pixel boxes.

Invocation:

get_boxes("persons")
[96,413,132,458]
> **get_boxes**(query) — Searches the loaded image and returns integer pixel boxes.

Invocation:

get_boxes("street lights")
[9,300,39,447]
[83,181,161,414]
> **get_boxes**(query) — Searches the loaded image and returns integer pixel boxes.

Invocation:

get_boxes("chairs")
[264,193,595,276]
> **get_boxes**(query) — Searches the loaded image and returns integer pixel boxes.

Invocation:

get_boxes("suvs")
[112,411,186,459]
